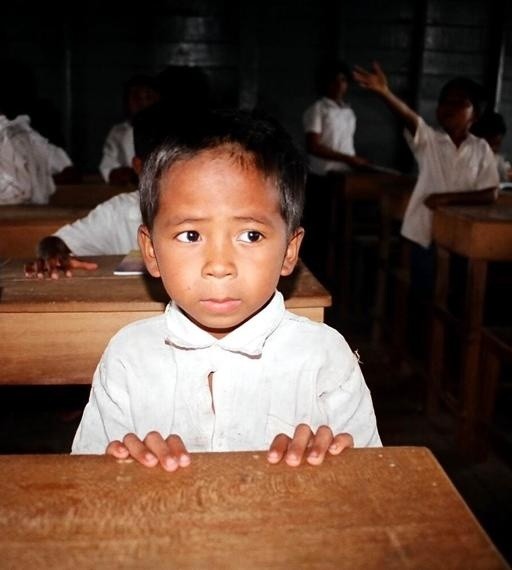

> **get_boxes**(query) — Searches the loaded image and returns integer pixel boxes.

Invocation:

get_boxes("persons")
[62,106,387,474]
[0,58,512,396]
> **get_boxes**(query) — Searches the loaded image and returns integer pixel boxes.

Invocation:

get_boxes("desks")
[1,446,510,570]
[335,166,512,401]
[2,169,133,258]
[1,254,330,386]
